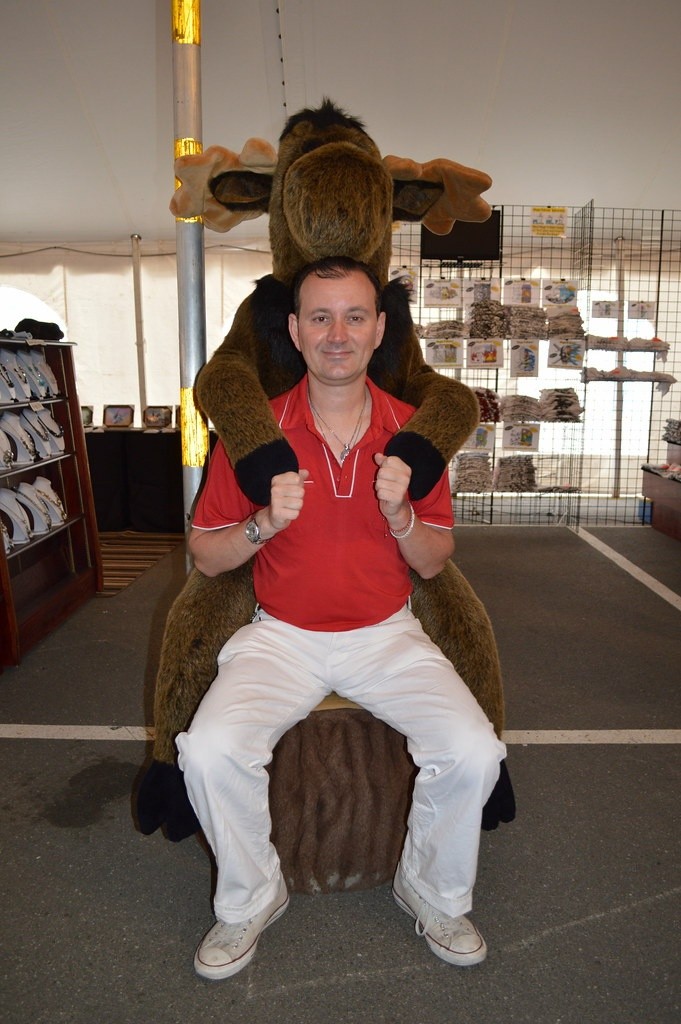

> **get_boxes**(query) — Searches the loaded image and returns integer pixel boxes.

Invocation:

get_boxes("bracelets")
[383,501,416,539]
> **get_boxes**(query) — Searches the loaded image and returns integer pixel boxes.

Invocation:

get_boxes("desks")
[84,426,217,533]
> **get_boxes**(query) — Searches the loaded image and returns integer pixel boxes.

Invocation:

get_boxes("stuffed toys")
[134,97,516,842]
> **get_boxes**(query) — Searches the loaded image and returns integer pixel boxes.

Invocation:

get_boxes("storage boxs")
[636,493,653,525]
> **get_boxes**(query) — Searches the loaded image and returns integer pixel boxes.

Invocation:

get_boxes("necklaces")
[0,354,68,550]
[307,385,367,464]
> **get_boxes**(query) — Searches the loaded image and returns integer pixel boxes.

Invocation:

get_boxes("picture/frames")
[140,405,173,428]
[80,405,94,427]
[103,404,135,428]
[175,405,181,428]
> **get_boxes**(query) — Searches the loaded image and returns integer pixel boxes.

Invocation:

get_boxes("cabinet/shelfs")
[641,439,681,541]
[0,339,104,668]
[422,276,681,497]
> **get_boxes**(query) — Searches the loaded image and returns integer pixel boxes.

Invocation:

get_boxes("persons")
[174,255,507,980]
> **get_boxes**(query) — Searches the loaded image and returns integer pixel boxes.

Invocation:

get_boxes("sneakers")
[391,865,489,967]
[194,870,292,981]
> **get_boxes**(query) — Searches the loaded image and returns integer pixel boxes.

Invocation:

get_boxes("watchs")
[245,511,271,545]
